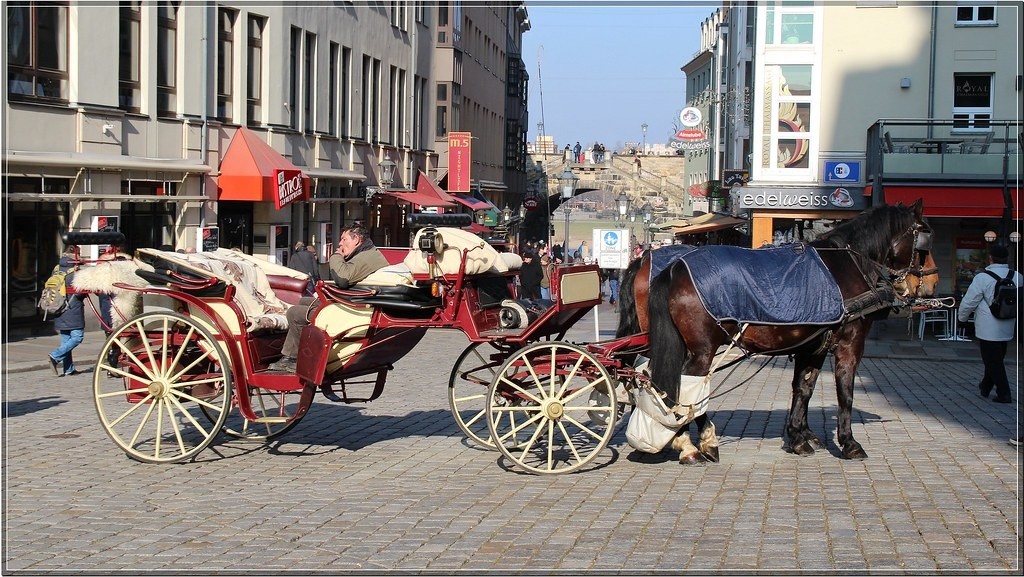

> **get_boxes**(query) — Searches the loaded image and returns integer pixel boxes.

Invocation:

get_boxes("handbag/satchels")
[309,274,321,286]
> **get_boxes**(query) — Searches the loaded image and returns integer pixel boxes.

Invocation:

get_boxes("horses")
[613,196,938,468]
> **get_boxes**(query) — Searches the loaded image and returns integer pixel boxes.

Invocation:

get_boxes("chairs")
[920,309,949,340]
[960,132,994,153]
[880,132,911,152]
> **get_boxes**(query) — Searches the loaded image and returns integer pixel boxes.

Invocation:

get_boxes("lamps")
[517,202,527,220]
[376,150,397,188]
[501,203,513,222]
[1010,231,1021,243]
[985,231,996,243]
[475,209,487,224]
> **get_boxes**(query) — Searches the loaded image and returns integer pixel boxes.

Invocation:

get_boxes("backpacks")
[37,264,77,321]
[981,269,1017,319]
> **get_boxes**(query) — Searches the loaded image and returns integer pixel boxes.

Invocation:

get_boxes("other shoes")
[611,301,619,309]
[992,396,1011,403]
[68,370,82,376]
[1010,436,1024,446]
[48,355,59,375]
[979,383,988,398]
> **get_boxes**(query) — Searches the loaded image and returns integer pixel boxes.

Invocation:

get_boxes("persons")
[289,242,320,295]
[958,245,1021,404]
[48,244,197,379]
[561,141,677,163]
[266,223,390,370]
[505,234,682,308]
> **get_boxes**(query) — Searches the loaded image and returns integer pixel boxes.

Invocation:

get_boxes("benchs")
[314,265,417,331]
[167,257,290,333]
[425,221,522,286]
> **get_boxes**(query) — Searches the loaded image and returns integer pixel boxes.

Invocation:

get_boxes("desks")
[941,303,969,341]
[911,142,946,153]
[921,137,963,153]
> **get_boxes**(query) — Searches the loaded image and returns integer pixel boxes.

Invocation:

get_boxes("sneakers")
[267,356,296,373]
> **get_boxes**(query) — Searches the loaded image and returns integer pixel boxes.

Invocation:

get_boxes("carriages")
[61,195,936,478]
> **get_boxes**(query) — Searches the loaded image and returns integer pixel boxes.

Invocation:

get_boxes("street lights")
[641,120,648,155]
[558,162,580,264]
[614,189,652,255]
[536,121,543,153]
[983,204,1021,273]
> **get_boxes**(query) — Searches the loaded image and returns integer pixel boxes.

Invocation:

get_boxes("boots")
[107,350,123,378]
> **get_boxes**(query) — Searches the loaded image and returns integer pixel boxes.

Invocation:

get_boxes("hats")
[522,254,535,259]
[990,245,1009,258]
[556,254,564,261]
[542,255,548,262]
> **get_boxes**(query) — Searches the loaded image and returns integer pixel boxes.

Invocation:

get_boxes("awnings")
[648,211,747,236]
[388,190,493,211]
[864,185,1024,219]
